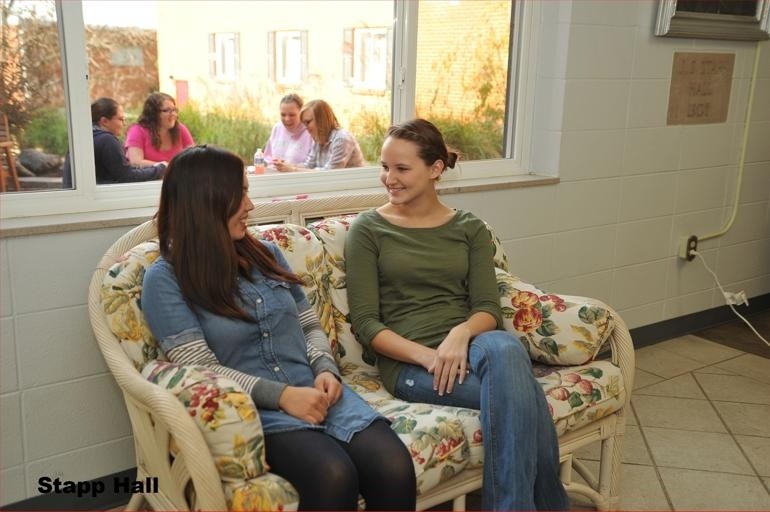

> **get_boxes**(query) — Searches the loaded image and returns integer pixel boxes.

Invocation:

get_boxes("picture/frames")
[655,0,769,44]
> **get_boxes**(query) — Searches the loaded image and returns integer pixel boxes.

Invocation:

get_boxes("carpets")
[693,302,770,359]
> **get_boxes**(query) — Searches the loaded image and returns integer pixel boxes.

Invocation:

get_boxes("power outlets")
[680,232,700,262]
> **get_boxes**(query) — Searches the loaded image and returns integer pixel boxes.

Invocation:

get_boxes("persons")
[62,98,167,190]
[270,99,366,172]
[262,95,312,167]
[136,145,418,512]
[346,119,570,512]
[126,92,196,166]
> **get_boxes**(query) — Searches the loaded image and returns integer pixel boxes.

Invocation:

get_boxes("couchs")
[87,192,636,512]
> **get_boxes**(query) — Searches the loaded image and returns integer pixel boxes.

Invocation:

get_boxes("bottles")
[253,146,264,175]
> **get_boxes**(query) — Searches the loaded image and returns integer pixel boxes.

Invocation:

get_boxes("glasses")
[303,118,313,125]
[160,107,179,113]
